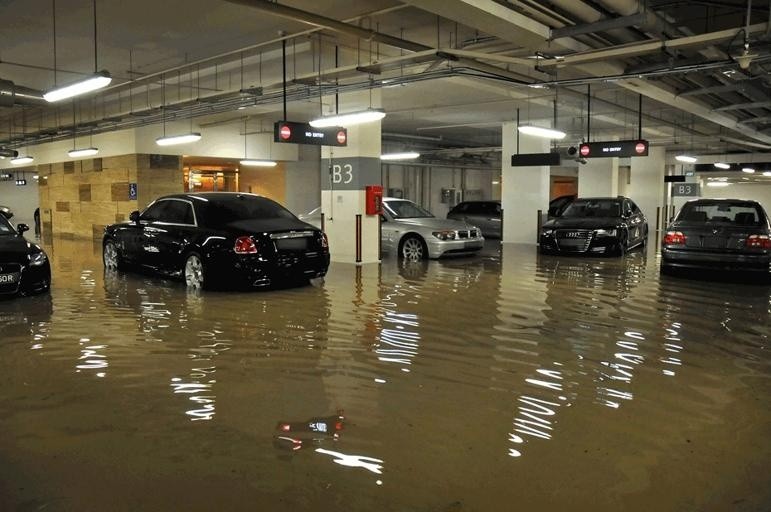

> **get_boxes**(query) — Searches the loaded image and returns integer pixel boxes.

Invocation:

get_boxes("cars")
[659,199,771,276]
[102,190,330,291]
[549,196,577,217]
[446,200,557,238]
[539,196,648,259]
[300,196,485,261]
[0,207,53,301]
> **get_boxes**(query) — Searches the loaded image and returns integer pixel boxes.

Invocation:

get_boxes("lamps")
[43,0,112,103]
[308,30,387,128]
[8,66,280,168]
[517,68,567,140]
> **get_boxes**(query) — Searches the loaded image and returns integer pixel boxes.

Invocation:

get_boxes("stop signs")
[280,125,291,139]
[336,131,346,143]
[636,143,646,153]
[581,146,589,156]
